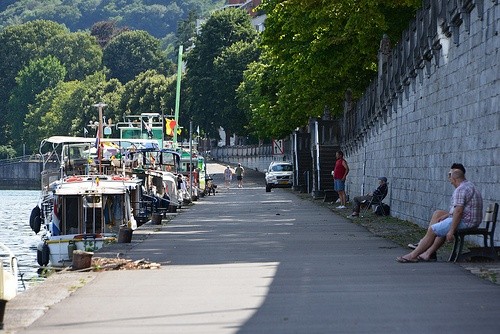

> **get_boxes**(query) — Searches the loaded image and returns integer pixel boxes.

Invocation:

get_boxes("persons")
[351,177,388,216]
[224,166,232,191]
[333,150,349,209]
[235,163,244,188]
[393,163,483,262]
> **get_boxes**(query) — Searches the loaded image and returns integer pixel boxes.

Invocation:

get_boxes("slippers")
[417,255,429,262]
[393,256,418,263]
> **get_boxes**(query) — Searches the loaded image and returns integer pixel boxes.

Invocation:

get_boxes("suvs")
[263,161,293,192]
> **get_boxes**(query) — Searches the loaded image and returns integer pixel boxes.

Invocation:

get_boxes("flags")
[52,201,60,236]
[95,128,99,149]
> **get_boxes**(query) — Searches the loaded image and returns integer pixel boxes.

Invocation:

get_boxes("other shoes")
[429,254,437,261]
[347,212,359,219]
[335,205,347,209]
[408,242,418,249]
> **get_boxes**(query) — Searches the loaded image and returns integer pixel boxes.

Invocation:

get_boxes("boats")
[39,113,206,268]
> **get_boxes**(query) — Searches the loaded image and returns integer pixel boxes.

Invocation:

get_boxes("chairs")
[447,203,499,262]
[362,182,388,217]
